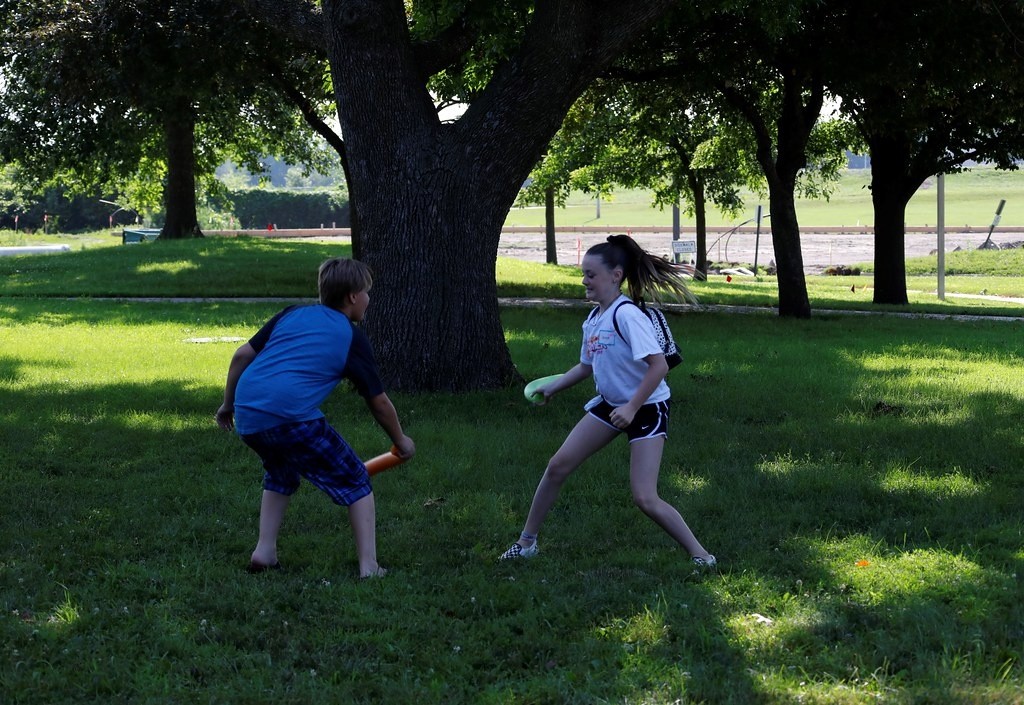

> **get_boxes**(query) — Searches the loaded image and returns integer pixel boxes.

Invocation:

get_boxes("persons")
[497,234,716,576]
[213,256,417,576]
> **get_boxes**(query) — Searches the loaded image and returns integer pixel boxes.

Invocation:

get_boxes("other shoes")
[498,539,537,560]
[250,560,283,572]
[361,566,388,579]
[692,555,717,574]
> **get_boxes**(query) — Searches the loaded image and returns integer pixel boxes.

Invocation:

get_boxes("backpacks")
[612,296,684,370]
